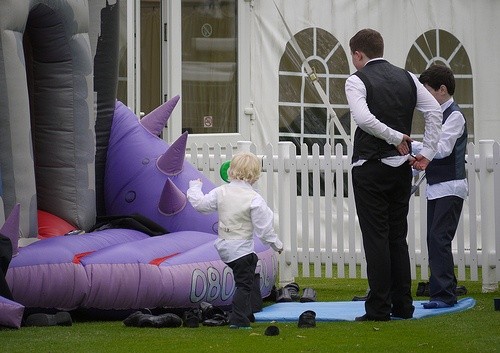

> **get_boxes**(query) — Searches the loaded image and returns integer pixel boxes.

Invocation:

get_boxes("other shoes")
[200,302,228,319]
[275,287,293,302]
[26,311,73,327]
[184,307,206,328]
[300,287,317,301]
[205,314,231,326]
[283,282,300,299]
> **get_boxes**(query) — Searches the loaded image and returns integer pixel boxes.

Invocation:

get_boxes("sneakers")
[137,312,183,328]
[124,307,153,327]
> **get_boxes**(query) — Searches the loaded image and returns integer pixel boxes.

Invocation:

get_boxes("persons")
[345,29,443,322]
[187,152,283,331]
[412,65,469,308]
